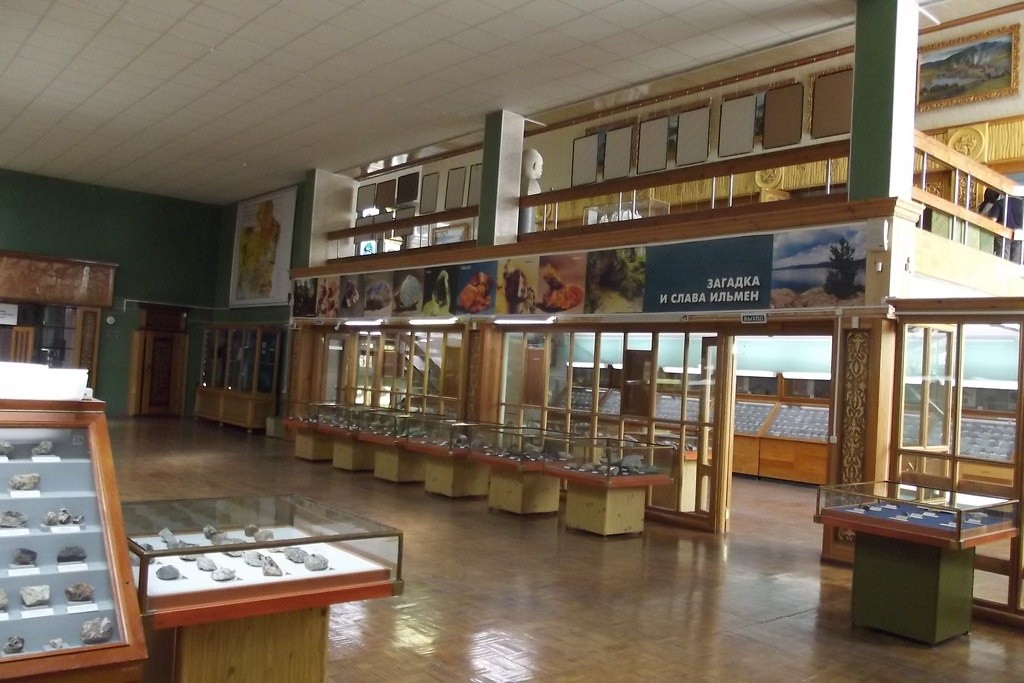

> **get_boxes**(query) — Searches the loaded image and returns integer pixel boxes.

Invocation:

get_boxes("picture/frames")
[228,185,298,309]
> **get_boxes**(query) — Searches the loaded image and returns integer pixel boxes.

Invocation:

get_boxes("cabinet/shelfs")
[193,322,282,433]
[0,398,150,683]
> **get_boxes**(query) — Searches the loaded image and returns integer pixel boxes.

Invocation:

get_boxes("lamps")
[344,319,383,326]
[408,316,459,325]
[494,316,558,325]
